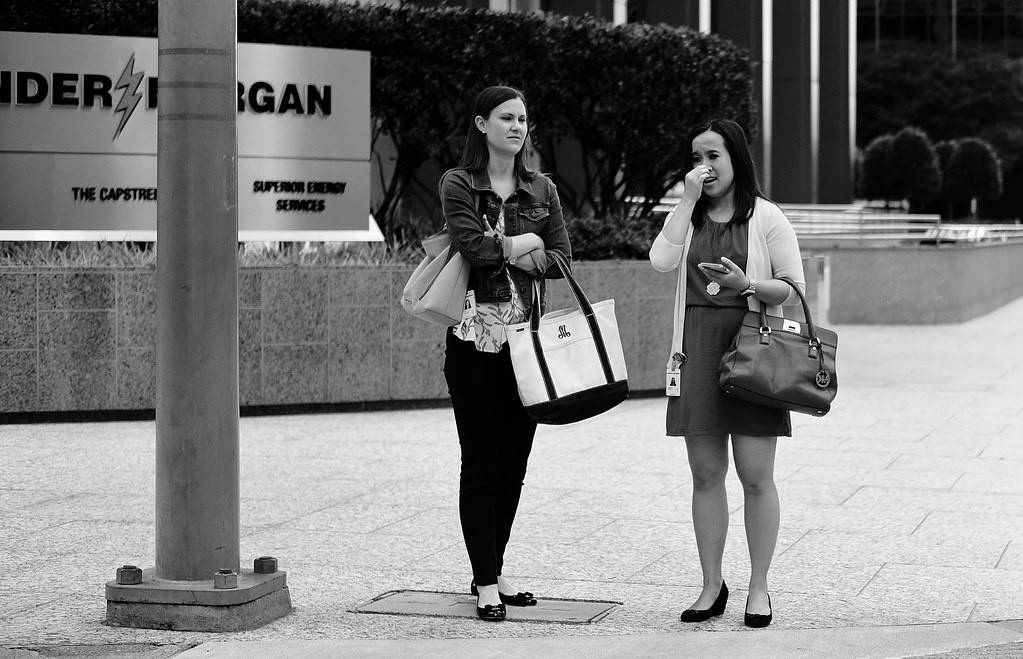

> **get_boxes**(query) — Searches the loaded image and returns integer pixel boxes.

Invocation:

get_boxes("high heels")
[745,593,772,627]
[681,580,729,622]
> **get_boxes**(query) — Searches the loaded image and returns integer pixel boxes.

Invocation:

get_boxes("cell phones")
[700,262,731,275]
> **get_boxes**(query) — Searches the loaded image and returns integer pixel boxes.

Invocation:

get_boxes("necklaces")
[705,216,727,296]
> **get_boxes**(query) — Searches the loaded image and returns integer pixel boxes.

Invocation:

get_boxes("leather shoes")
[477,596,507,620]
[471,579,538,606]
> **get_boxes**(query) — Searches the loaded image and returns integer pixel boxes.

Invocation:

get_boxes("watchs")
[740,276,756,298]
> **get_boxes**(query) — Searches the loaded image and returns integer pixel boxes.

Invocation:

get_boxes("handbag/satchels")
[504,251,628,425]
[399,185,488,328]
[718,278,839,416]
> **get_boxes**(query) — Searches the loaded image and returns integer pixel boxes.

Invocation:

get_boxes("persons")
[648,119,805,629]
[437,86,573,623]
